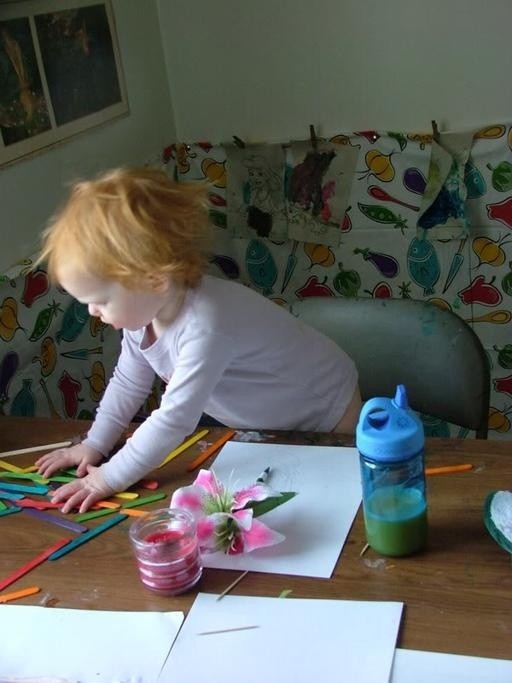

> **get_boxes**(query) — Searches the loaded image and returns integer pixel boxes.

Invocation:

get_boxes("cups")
[128,507,207,600]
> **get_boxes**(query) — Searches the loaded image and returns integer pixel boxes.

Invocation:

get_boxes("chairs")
[265,295,492,442]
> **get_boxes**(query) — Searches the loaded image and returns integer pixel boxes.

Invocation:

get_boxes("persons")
[28,162,365,517]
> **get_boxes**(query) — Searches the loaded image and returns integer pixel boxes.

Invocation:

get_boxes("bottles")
[349,380,435,560]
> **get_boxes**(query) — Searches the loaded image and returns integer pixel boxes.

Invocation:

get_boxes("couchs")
[2,125,509,443]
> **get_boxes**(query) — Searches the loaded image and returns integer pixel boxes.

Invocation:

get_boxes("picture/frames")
[1,0,131,171]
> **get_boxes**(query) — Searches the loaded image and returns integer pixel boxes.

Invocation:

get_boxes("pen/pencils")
[256,467,270,482]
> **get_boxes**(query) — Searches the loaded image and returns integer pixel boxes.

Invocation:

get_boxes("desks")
[0,413,510,679]
